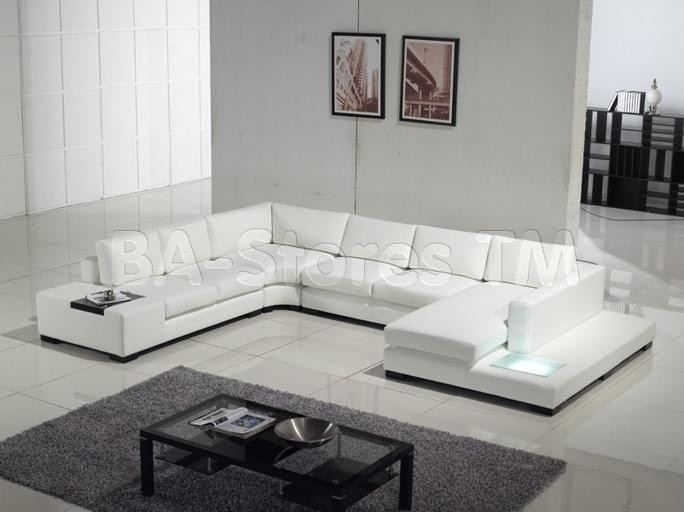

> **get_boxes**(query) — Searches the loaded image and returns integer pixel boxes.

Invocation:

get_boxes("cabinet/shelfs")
[580,107,684,218]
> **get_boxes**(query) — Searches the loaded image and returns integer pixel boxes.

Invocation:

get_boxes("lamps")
[645,78,662,116]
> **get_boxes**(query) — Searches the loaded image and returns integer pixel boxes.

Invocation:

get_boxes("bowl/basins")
[275,417,341,447]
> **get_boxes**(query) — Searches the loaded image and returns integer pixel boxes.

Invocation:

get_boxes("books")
[607,89,646,116]
[190,406,276,440]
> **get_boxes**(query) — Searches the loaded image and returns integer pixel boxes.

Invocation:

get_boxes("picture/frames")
[331,31,386,119]
[399,35,459,126]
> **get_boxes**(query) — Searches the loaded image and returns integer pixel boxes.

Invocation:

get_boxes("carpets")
[2,365,568,512]
